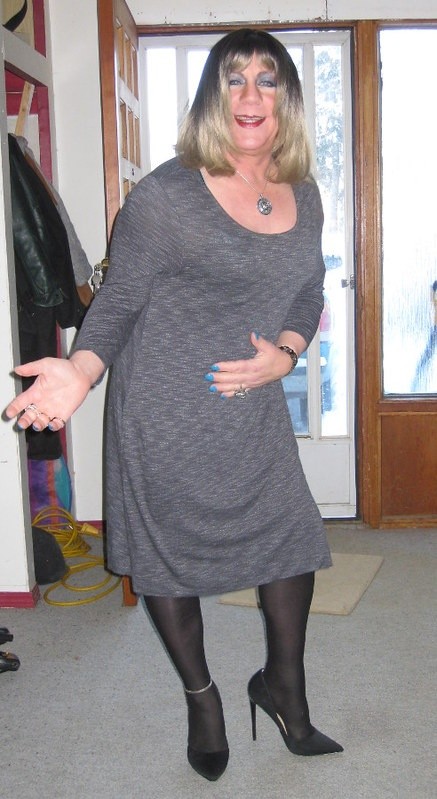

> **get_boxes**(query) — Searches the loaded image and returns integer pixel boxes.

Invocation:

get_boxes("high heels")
[183,683,229,782]
[245,668,344,757]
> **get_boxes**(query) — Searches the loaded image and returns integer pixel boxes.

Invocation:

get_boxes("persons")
[4,29,345,780]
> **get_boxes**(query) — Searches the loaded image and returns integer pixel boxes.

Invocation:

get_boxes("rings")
[24,404,41,416]
[235,385,246,399]
[54,417,65,424]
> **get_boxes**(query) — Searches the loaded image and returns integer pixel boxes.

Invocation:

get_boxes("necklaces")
[235,169,273,215]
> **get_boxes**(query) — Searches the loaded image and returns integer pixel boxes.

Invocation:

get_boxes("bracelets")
[279,345,298,372]
